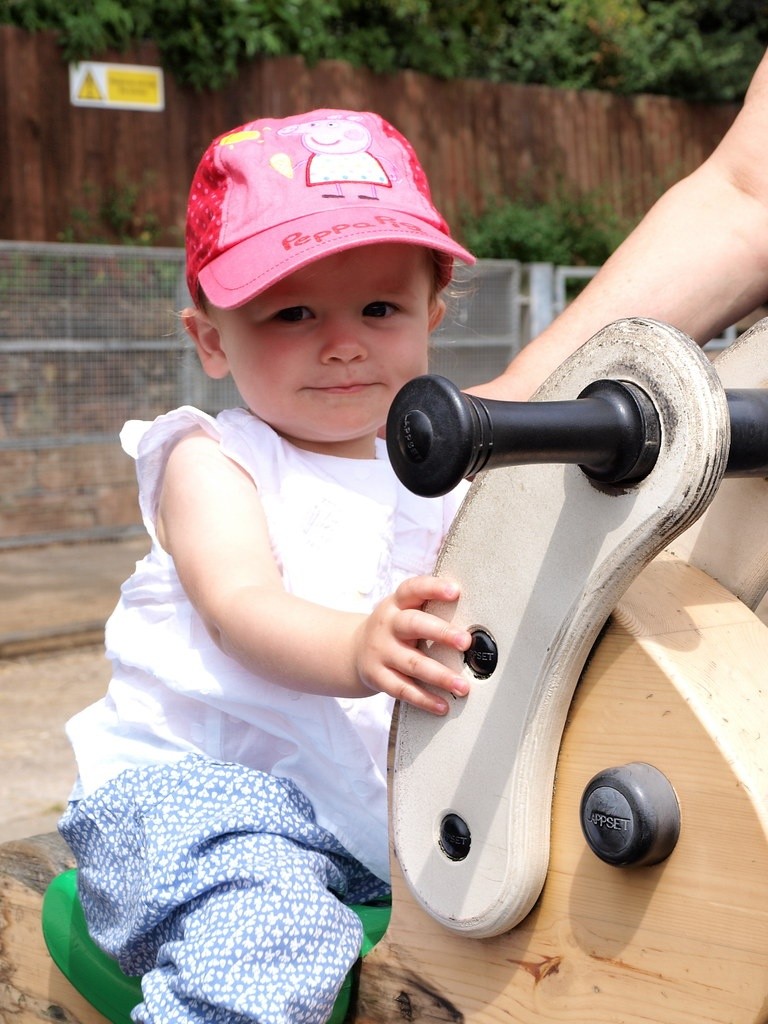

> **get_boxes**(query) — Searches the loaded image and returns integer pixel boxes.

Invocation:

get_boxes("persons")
[54,106,480,1024]
[452,46,767,403]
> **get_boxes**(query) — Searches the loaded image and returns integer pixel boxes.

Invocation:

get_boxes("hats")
[184,106,477,309]
